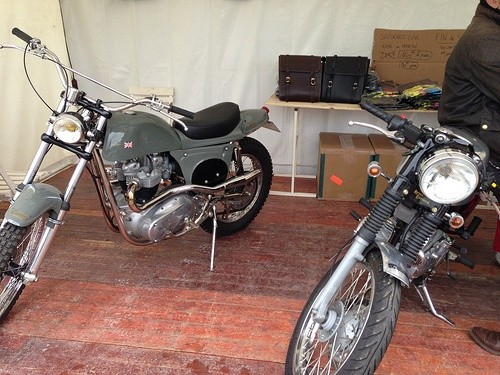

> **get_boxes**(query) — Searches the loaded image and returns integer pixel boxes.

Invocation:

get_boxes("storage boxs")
[368,133,412,202]
[317,132,376,203]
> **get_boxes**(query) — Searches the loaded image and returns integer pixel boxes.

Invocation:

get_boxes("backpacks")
[322,55,371,103]
[279,54,322,103]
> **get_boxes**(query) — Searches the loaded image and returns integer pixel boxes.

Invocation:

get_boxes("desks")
[266,91,438,199]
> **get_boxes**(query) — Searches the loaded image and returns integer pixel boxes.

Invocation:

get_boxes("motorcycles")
[284,99,497,375]
[2,27,279,322]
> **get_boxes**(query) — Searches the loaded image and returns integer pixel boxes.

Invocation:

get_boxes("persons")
[437,0,500,266]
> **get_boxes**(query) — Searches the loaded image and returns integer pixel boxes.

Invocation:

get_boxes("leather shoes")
[470,326,500,356]
